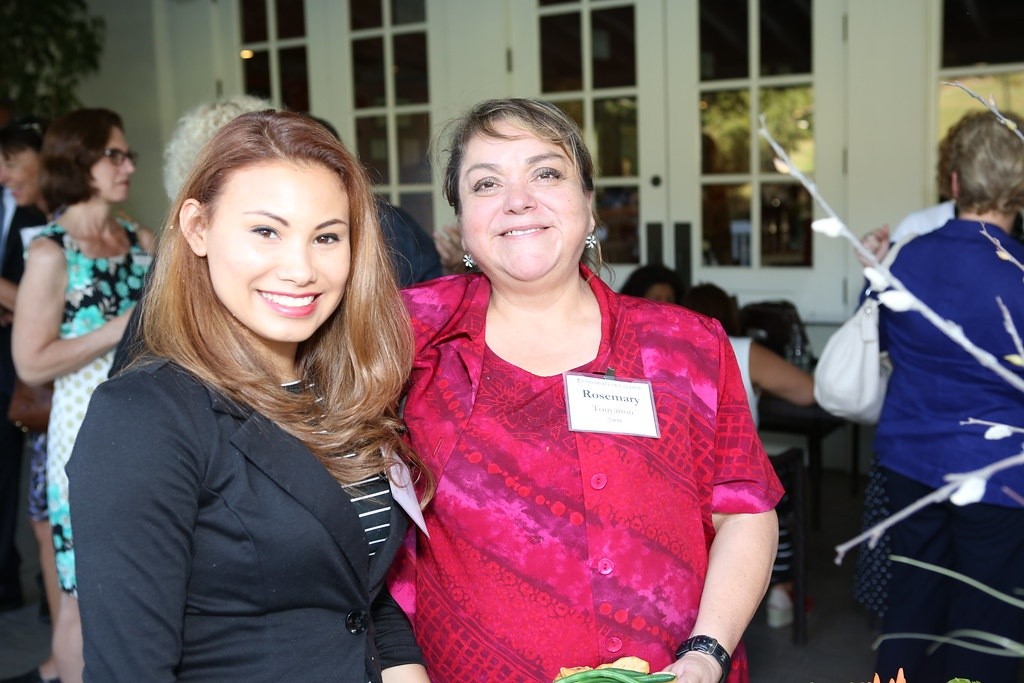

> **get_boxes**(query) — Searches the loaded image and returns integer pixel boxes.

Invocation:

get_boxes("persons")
[63,108,432,683]
[162,95,446,290]
[854,109,1024,683]
[387,98,786,683]
[616,261,814,433]
[0,107,158,683]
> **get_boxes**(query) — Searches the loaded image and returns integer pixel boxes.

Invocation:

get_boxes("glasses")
[104,147,140,165]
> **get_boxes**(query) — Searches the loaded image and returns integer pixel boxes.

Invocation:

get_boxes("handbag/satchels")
[812,285,894,426]
[7,375,54,433]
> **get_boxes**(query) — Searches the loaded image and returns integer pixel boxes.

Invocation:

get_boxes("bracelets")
[675,635,732,683]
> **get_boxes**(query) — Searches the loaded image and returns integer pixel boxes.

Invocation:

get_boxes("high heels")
[766,592,815,629]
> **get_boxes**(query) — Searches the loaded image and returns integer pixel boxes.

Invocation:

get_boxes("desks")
[758,377,861,534]
[758,443,809,647]
